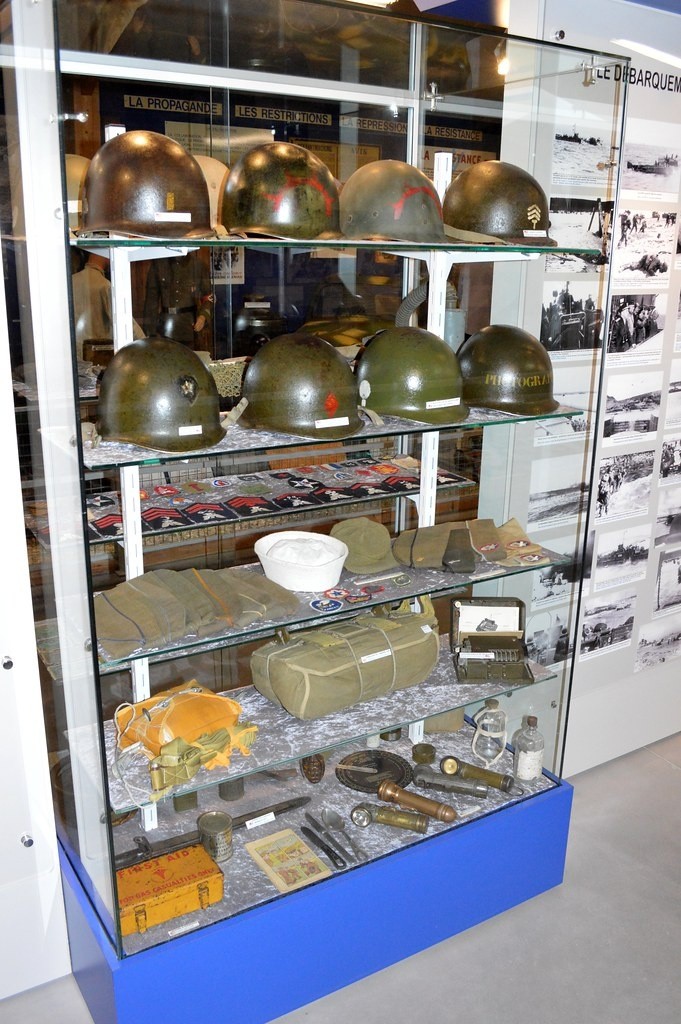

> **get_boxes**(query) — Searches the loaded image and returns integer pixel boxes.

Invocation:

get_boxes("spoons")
[323,809,368,861]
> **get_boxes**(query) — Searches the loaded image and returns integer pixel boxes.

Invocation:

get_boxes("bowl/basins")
[254,531,347,592]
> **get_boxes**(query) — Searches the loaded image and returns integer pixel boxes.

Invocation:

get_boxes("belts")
[162,307,195,313]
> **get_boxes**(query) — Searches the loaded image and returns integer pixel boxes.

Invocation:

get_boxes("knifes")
[301,828,347,870]
[305,813,356,865]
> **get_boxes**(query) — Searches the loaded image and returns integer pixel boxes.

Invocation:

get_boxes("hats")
[329,517,400,575]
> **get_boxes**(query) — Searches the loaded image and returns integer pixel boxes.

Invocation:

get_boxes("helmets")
[76,131,217,239]
[357,326,471,424]
[340,160,446,245]
[222,141,338,241]
[456,324,561,416]
[442,161,559,245]
[236,333,366,441]
[98,340,227,452]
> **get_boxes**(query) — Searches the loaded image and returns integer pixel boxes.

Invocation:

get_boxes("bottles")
[476,700,506,761]
[514,716,544,783]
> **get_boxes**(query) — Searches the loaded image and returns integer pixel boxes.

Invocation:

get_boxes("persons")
[611,297,659,352]
[619,253,667,278]
[597,466,628,517]
[661,443,674,477]
[664,214,676,228]
[584,295,595,312]
[617,210,647,248]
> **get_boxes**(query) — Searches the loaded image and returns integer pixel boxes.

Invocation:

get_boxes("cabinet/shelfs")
[0,0,631,1024]
[472,0,681,780]
